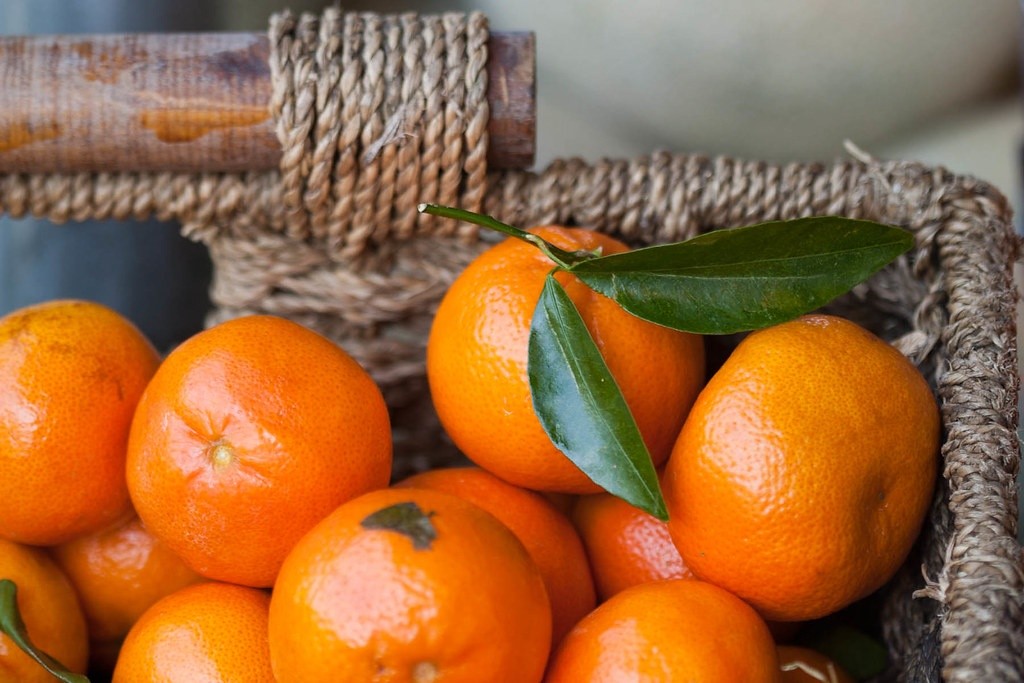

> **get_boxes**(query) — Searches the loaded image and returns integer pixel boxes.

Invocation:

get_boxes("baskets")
[0,9,1024,683]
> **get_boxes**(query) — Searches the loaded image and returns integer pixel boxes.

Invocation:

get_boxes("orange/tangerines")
[0,203,939,683]
